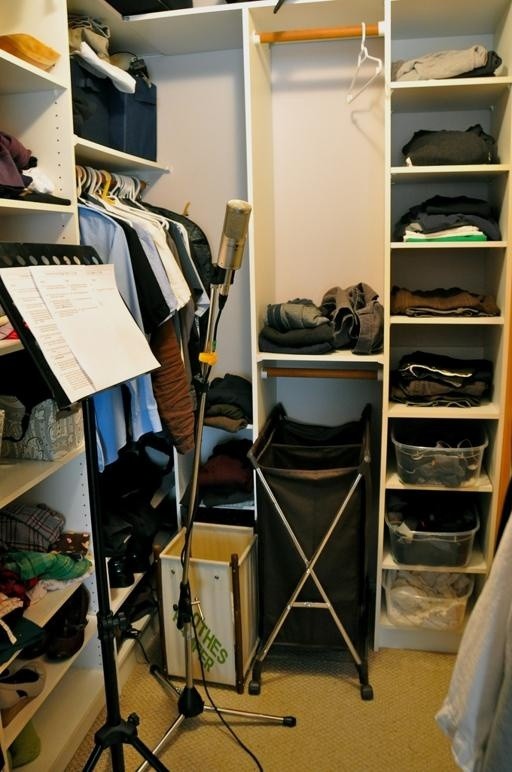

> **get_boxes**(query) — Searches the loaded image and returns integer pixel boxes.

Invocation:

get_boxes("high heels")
[16,582,90,664]
[0,660,47,710]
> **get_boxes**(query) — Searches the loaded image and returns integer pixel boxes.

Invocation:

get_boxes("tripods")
[138,284,296,772]
[82,396,170,772]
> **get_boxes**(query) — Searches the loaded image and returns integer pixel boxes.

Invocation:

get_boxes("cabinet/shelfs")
[1,2,512,772]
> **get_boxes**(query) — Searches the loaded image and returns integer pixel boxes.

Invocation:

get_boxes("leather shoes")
[108,544,150,588]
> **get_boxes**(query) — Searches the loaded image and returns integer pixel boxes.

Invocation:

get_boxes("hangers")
[345,21,383,107]
[73,164,194,236]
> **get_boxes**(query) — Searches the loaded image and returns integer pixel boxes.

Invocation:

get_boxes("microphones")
[219,199,252,309]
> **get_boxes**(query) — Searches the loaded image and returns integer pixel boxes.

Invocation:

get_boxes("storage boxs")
[70,59,157,161]
[380,430,490,633]
